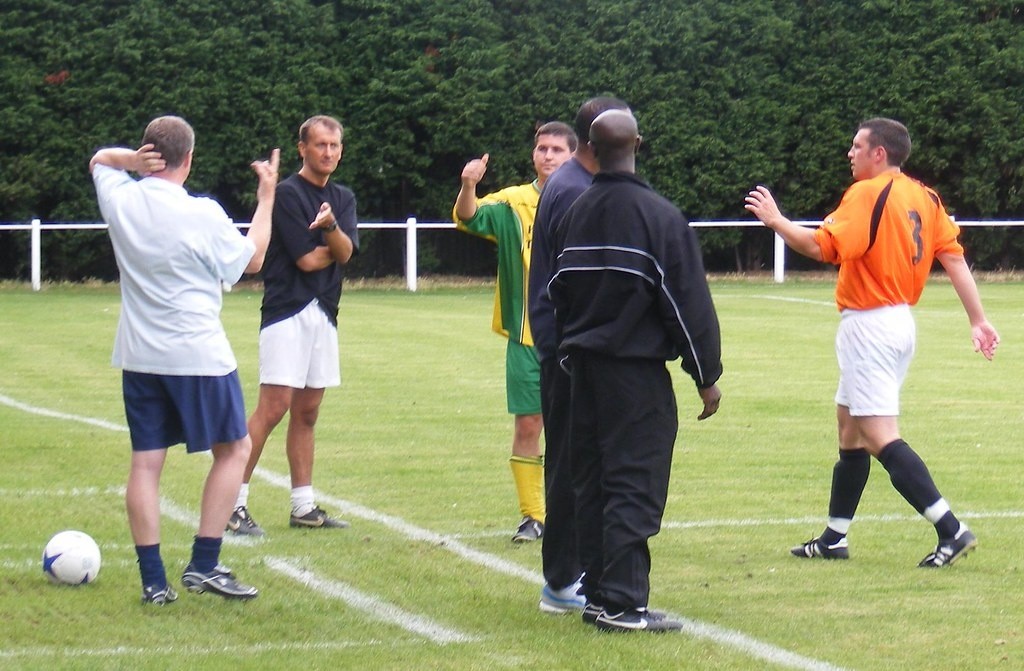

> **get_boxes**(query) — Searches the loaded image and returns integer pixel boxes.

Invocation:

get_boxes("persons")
[226,115,357,536]
[545,109,725,632]
[451,121,579,545]
[522,91,633,614]
[87,115,280,611]
[742,116,1000,568]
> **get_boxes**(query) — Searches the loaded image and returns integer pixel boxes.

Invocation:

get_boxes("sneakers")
[917,521,978,568]
[289,505,350,528]
[512,515,545,542]
[540,571,589,612]
[596,606,684,631]
[225,505,264,536]
[142,581,178,606]
[581,600,667,623]
[791,532,850,560]
[181,562,258,599]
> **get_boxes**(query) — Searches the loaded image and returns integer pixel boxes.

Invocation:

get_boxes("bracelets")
[321,221,337,232]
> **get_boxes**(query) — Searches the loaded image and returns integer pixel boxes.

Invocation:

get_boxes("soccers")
[41,529,102,588]
